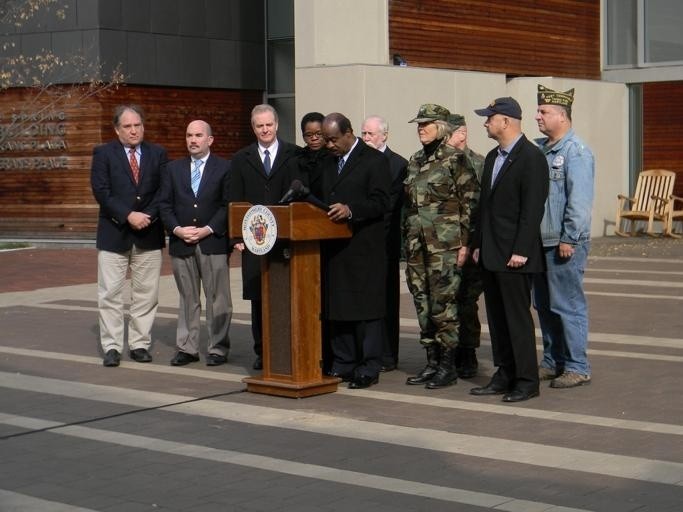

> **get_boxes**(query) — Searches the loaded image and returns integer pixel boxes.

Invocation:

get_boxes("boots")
[404,342,456,390]
[458,345,478,379]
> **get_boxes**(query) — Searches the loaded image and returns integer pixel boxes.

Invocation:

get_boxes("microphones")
[279,180,302,203]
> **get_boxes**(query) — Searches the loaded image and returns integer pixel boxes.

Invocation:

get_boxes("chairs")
[663,195,683,237]
[615,168,675,237]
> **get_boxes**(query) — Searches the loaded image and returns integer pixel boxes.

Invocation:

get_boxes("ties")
[128,147,139,184]
[262,150,273,174]
[336,156,345,175]
[191,160,204,197]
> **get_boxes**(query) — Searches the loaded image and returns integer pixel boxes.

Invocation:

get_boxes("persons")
[398,103,485,388]
[469,96,549,402]
[229,103,303,369]
[158,119,234,366]
[89,103,168,367]
[288,112,409,387]
[530,83,596,390]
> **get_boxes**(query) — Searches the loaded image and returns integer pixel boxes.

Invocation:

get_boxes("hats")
[535,83,576,108]
[407,102,467,133]
[473,96,522,120]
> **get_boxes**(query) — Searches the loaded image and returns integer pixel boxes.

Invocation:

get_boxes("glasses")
[304,130,324,139]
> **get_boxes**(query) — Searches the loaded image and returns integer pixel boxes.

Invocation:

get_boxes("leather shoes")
[205,354,228,367]
[129,349,152,363]
[470,382,510,397]
[349,372,381,389]
[377,363,398,373]
[170,351,200,366]
[103,347,123,368]
[501,390,542,401]
[253,357,262,370]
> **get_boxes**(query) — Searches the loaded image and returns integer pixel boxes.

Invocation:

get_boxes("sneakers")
[538,363,558,379]
[550,369,592,390]
